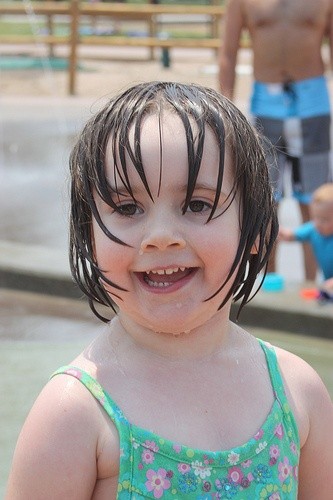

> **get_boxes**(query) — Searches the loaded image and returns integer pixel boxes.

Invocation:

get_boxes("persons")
[275,182,333,301]
[219,0,332,289]
[7,81,332,500]
